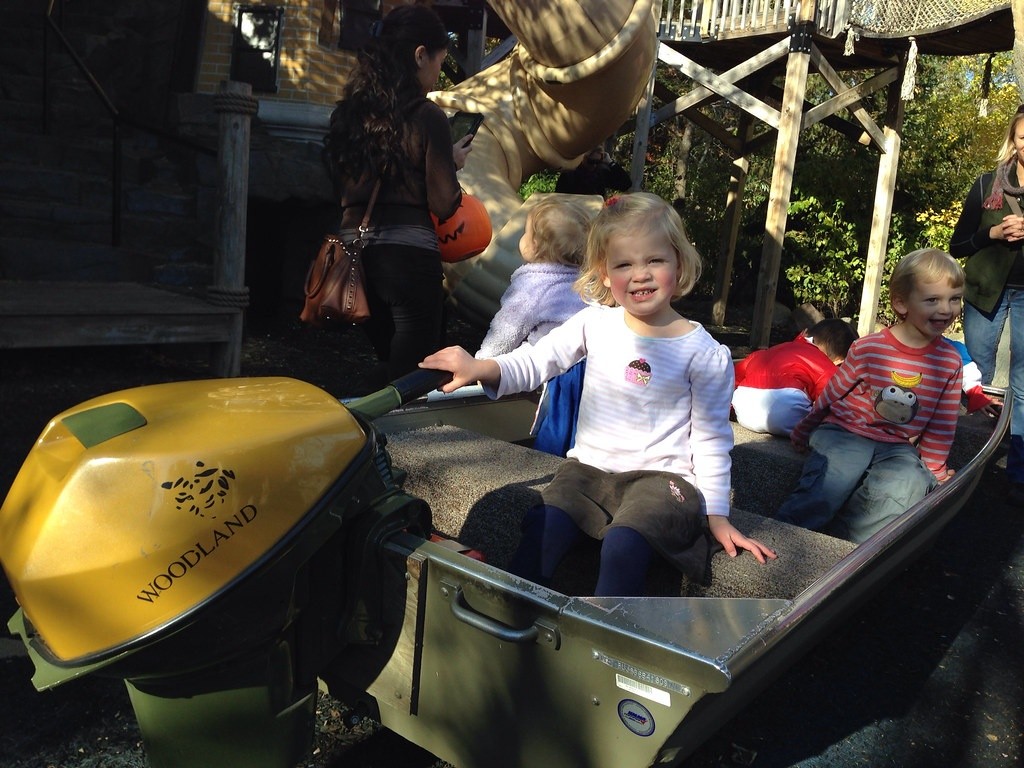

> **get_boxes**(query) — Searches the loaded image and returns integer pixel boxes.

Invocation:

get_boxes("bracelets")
[608,161,615,166]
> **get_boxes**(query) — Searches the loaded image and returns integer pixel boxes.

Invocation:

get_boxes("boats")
[1,358,1014,768]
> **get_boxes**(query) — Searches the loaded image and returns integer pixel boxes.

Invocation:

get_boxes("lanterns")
[431,194,491,262]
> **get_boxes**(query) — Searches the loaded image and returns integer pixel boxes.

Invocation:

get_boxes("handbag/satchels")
[300,234,371,326]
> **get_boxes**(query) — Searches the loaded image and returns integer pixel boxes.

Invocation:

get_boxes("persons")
[418,191,777,599]
[474,196,593,359]
[950,104,1024,510]
[329,6,473,387]
[941,335,1003,416]
[732,318,860,437]
[788,248,967,544]
[555,145,631,199]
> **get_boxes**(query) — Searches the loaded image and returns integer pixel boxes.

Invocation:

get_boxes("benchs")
[379,423,858,613]
[0,283,234,375]
[947,414,996,472]
[724,420,849,544]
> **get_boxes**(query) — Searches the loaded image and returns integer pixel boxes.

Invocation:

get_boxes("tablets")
[451,111,484,149]
